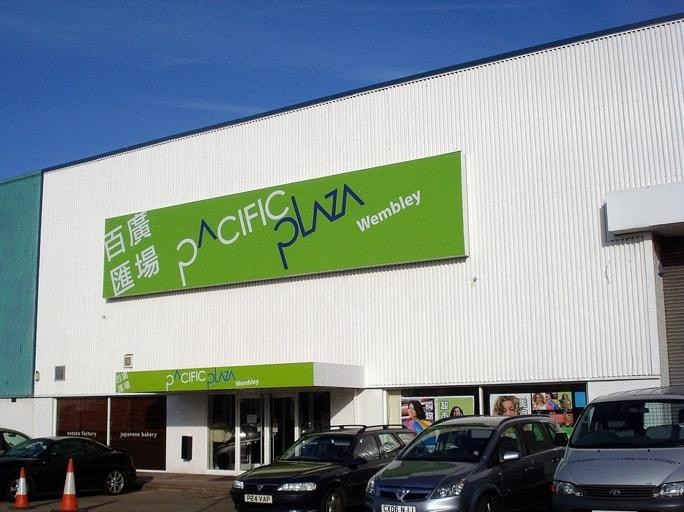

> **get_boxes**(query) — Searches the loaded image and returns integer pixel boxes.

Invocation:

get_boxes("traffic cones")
[50,460,89,512]
[7,466,37,511]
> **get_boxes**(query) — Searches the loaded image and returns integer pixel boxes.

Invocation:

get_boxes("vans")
[366,414,568,512]
[550,384,684,512]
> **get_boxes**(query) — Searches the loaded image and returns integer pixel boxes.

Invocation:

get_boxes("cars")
[210,421,263,470]
[0,428,138,503]
[229,423,430,512]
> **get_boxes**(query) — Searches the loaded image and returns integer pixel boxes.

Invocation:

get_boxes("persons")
[492,395,529,434]
[544,392,560,410]
[407,399,431,435]
[532,393,546,410]
[449,406,464,417]
[556,392,572,407]
